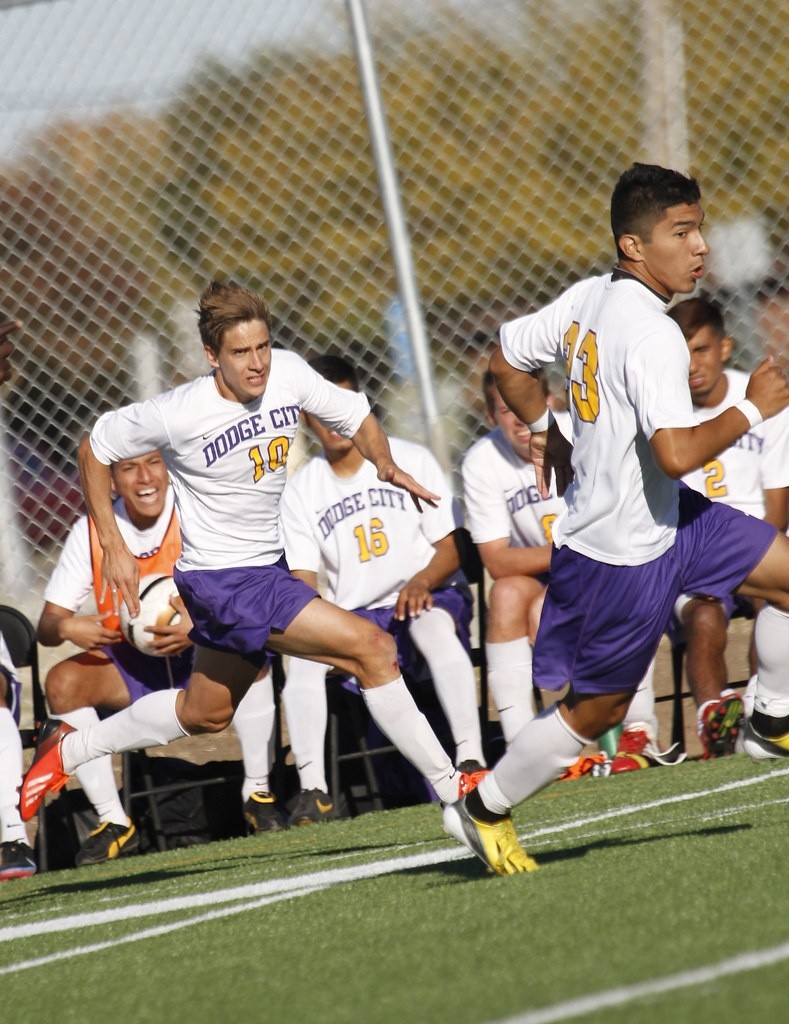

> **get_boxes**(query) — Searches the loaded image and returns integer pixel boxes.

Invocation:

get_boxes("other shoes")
[556,752,606,781]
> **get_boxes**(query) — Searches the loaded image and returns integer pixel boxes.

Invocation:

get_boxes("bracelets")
[528,408,555,433]
[732,401,764,428]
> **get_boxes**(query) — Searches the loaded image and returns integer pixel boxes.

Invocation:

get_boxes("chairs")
[0,527,755,871]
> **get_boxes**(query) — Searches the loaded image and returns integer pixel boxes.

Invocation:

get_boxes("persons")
[461,365,662,781]
[441,163,789,878]
[17,282,493,821]
[37,452,289,866]
[0,630,37,879]
[662,298,789,761]
[288,358,493,826]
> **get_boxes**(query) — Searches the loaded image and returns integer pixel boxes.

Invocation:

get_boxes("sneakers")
[75,816,140,868]
[16,717,79,822]
[438,770,491,810]
[287,786,335,827]
[242,791,288,837]
[0,838,38,880]
[608,724,655,775]
[441,795,539,877]
[735,715,789,759]
[456,758,485,774]
[698,688,746,761]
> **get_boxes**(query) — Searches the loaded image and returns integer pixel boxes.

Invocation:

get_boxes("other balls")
[121,573,186,658]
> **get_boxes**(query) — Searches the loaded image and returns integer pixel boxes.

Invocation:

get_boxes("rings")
[111,589,118,593]
